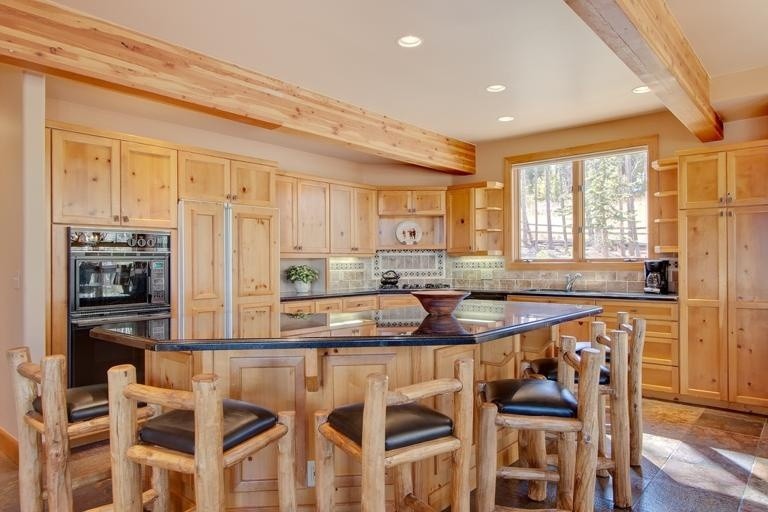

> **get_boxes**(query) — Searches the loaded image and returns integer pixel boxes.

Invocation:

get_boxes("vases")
[294,280,311,292]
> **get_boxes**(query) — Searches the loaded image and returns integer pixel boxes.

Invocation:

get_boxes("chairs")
[475,334,600,511]
[313,356,474,512]
[519,309,646,509]
[8,347,155,512]
[108,363,298,512]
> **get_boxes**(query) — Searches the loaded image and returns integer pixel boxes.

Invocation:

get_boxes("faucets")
[565,273,583,292]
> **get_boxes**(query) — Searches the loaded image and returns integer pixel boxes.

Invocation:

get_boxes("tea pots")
[381,270,400,284]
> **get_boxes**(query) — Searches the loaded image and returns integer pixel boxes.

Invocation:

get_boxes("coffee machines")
[644,257,668,294]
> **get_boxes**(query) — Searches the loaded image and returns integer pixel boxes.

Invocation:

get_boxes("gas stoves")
[377,283,450,292]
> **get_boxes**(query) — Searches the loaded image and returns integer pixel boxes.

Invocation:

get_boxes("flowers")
[287,265,318,281]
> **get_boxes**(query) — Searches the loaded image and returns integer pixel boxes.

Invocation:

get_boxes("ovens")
[64,226,176,390]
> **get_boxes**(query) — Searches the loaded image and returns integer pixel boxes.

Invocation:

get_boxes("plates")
[395,220,422,245]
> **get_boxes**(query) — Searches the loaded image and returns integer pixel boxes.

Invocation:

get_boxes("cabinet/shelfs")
[184,301,281,337]
[45,118,181,228]
[278,171,504,258]
[652,140,768,417]
[506,294,679,402]
[179,143,280,306]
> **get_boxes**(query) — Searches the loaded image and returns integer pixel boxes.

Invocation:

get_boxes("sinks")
[529,289,600,296]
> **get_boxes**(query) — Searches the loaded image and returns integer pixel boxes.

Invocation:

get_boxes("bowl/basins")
[411,314,472,336]
[409,290,473,316]
